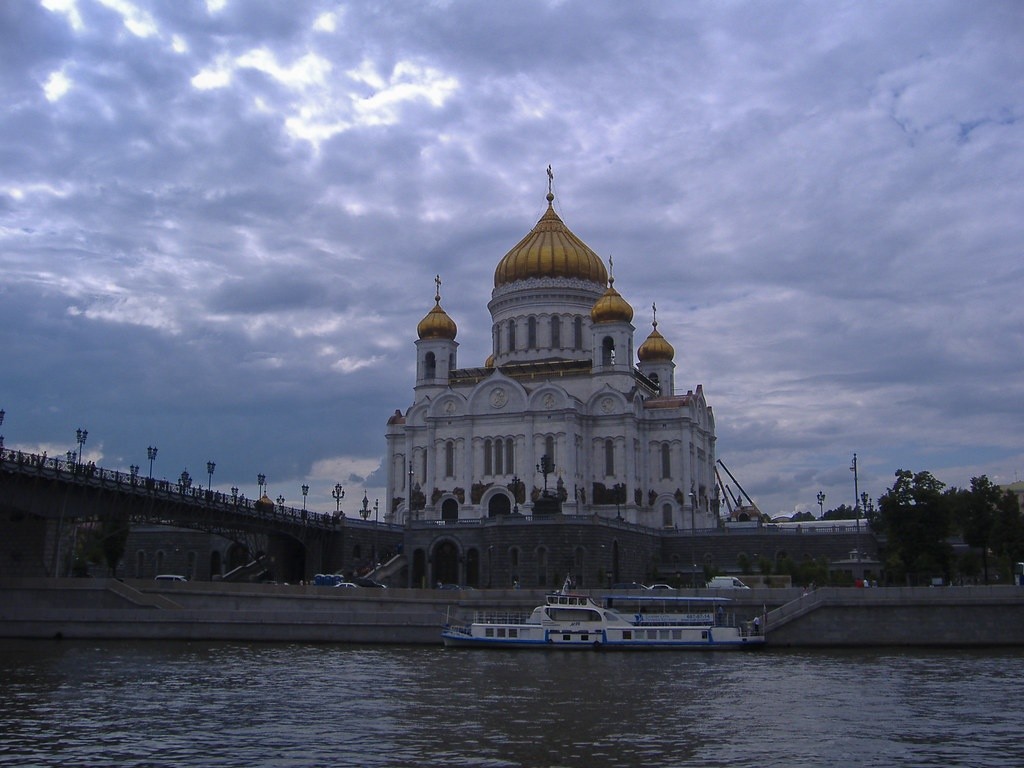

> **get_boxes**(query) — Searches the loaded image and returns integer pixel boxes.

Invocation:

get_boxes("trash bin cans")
[1013,563,1024,586]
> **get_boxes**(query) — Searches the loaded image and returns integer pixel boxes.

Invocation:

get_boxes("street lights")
[613,482,625,519]
[714,482,722,518]
[848,452,863,579]
[331,482,345,511]
[206,460,216,492]
[358,489,372,521]
[257,473,266,503]
[75,427,89,465]
[510,473,522,513]
[146,446,158,480]
[408,460,414,528]
[688,482,696,534]
[535,454,557,497]
[301,483,310,510]
[372,498,379,527]
[816,490,826,521]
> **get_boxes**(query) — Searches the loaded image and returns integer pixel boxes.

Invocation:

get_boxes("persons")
[512,576,520,589]
[239,494,245,503]
[299,578,315,586]
[800,585,809,596]
[871,579,878,588]
[753,615,761,636]
[854,577,863,587]
[811,576,817,590]
[862,579,870,588]
[746,619,752,637]
[397,539,402,554]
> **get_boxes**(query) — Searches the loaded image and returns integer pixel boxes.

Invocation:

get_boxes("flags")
[566,576,572,589]
[764,607,768,621]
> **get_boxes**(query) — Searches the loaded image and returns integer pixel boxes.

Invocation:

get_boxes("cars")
[437,583,473,591]
[647,584,677,589]
[611,582,648,589]
[155,574,190,581]
[333,582,359,588]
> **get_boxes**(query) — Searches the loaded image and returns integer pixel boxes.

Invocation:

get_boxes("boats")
[440,572,767,652]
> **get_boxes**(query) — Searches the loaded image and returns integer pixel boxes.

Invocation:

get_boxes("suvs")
[351,578,387,588]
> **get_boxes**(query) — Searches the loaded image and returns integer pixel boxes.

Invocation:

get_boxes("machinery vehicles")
[713,458,772,523]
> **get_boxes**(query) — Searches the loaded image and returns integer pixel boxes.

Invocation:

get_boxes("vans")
[704,575,750,589]
